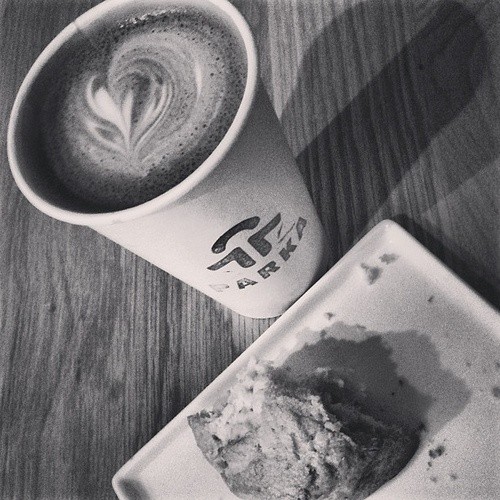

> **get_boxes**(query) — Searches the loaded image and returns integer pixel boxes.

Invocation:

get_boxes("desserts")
[186,359,420,500]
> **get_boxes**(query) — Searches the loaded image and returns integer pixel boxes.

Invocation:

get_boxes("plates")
[109,221,500,500]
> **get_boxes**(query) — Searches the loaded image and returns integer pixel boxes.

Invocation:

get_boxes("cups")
[5,0,331,319]
